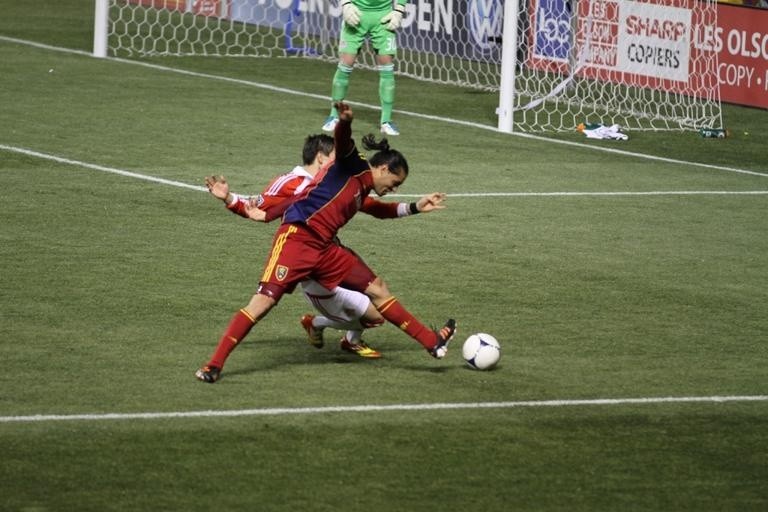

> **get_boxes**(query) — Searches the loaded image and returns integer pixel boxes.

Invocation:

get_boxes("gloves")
[380,10,403,31]
[342,3,362,26]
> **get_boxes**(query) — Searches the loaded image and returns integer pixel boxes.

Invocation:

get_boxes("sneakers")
[428,319,457,359]
[301,314,324,349]
[322,116,339,131]
[195,366,220,382]
[341,335,381,358]
[381,122,400,135]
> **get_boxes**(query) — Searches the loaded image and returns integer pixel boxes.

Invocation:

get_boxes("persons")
[195,98,457,381]
[203,132,382,359]
[319,0,405,136]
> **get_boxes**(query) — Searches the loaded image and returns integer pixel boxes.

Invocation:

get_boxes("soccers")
[463,332,501,370]
